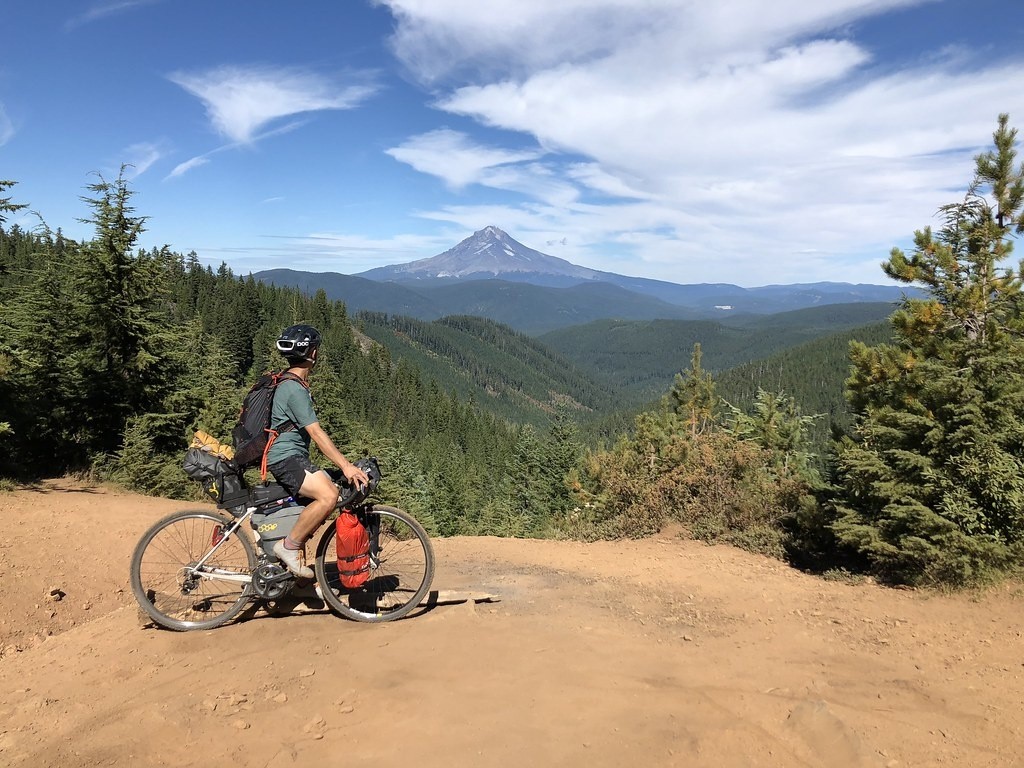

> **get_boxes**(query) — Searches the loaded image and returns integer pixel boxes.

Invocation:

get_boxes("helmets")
[276,324,324,359]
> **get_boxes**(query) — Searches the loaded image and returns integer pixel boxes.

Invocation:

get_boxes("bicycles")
[129,456,435,632]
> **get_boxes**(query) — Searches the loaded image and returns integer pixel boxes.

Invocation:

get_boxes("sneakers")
[272,538,314,578]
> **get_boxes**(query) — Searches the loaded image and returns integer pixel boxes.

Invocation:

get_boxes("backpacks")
[231,367,310,467]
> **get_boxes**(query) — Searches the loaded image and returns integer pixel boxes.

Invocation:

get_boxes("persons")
[264,324,369,579]
